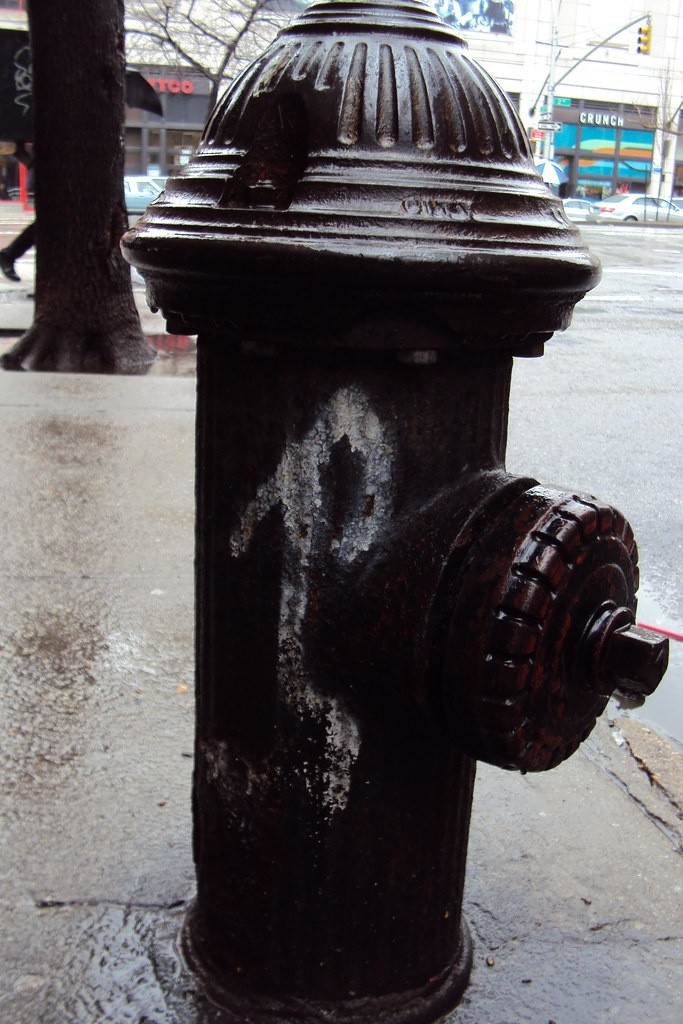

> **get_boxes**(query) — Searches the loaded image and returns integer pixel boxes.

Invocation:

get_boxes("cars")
[661,197,683,211]
[561,197,595,224]
[124,175,172,215]
[588,193,683,226]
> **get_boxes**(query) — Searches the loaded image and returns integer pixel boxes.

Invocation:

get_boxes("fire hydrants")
[112,1,672,1024]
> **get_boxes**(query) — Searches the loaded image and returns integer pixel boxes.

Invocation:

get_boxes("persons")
[0,141,36,283]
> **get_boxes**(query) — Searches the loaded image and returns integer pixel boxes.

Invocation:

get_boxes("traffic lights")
[636,25,651,54]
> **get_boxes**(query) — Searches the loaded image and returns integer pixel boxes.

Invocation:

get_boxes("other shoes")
[0,249,21,281]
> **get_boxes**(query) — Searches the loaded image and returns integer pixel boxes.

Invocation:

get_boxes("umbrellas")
[534,158,569,186]
[126,66,164,119]
